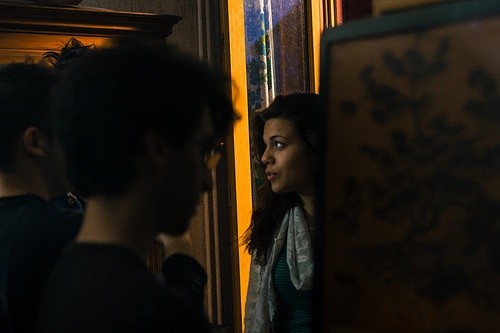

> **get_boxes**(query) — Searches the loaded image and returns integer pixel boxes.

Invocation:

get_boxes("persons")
[235,93,350,333]
[53,46,234,333]
[0,62,74,333]
